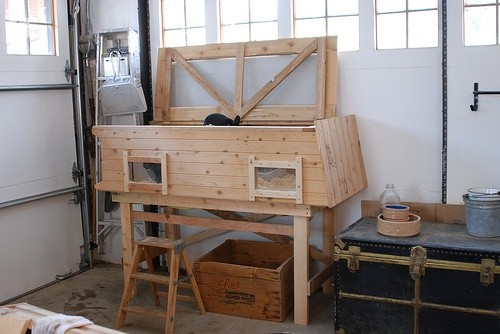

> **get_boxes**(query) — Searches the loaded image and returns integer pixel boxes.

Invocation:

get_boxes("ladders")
[115,237,205,334]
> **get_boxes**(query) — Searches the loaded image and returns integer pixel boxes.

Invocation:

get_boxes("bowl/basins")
[255,168,287,181]
[376,204,421,237]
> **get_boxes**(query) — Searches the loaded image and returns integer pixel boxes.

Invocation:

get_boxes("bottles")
[378,184,401,215]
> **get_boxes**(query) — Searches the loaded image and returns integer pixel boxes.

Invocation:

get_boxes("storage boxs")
[333,216,500,334]
[191,238,294,323]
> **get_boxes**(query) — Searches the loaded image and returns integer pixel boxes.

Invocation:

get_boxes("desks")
[110,192,337,327]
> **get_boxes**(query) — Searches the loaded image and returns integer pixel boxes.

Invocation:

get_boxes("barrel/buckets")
[462,187,500,237]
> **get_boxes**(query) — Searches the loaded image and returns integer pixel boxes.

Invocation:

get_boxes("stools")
[117,237,207,334]
